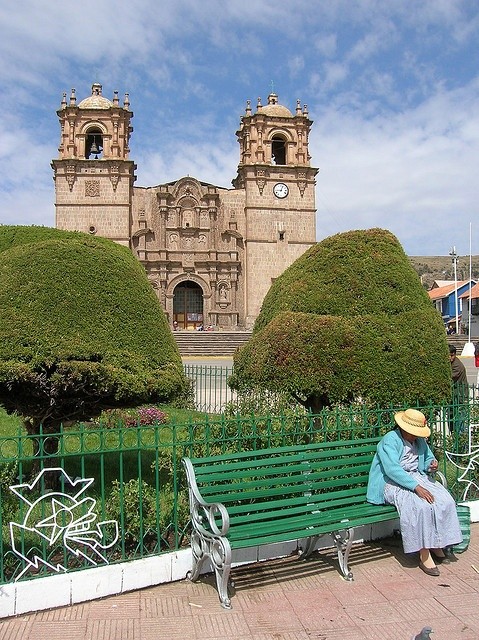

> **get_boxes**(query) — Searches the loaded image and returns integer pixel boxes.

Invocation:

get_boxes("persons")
[447,324,455,335]
[473,342,479,389]
[366,409,463,576]
[195,324,223,332]
[448,345,469,435]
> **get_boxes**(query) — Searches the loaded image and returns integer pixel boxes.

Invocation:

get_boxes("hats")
[394,408,430,437]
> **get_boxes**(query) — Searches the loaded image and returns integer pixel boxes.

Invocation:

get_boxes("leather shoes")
[419,559,439,575]
[432,550,450,564]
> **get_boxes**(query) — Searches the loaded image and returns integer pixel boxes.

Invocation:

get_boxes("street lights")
[449,246,459,333]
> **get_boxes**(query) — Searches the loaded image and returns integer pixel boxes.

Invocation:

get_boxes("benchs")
[179,450,448,610]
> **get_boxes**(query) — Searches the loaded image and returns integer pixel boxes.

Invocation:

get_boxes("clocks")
[273,182,289,199]
